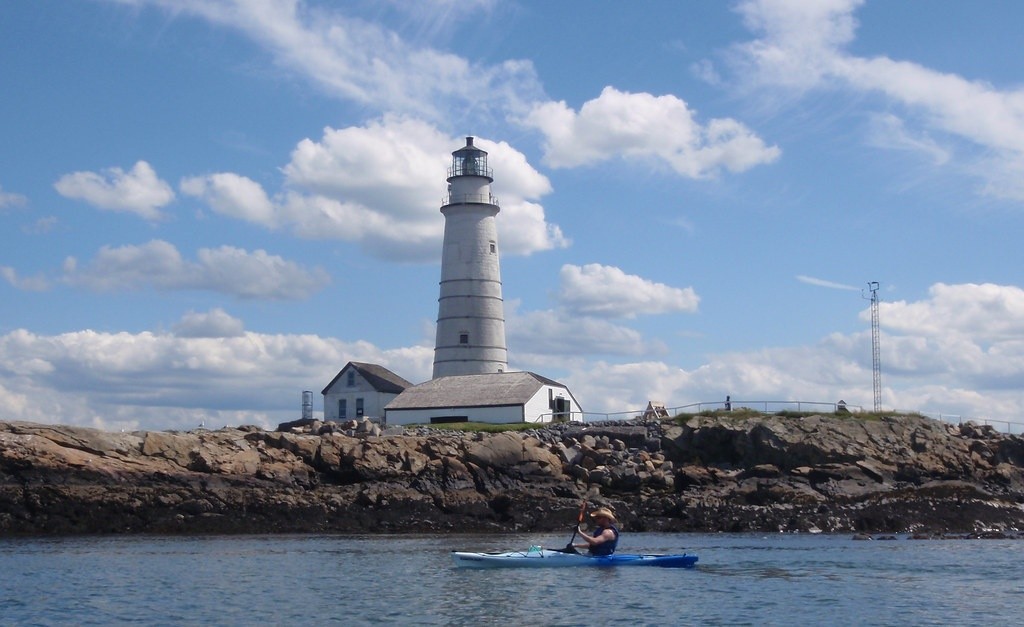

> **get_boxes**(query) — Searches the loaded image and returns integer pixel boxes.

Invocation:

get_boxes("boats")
[450,544,701,570]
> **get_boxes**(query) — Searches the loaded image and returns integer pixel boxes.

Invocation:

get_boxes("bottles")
[529,545,544,551]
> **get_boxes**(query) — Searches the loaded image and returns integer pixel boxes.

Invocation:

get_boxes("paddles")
[570,502,588,544]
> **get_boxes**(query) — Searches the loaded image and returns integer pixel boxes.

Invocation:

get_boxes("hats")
[590,507,615,520]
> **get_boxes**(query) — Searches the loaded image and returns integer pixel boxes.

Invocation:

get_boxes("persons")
[725,396,731,411]
[571,509,618,557]
